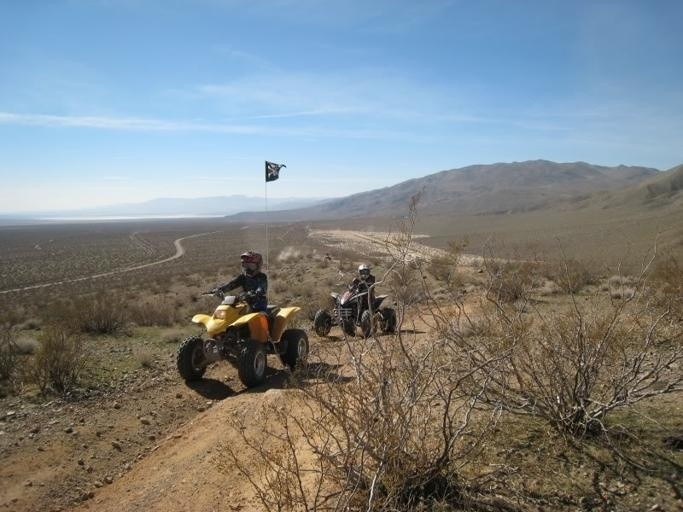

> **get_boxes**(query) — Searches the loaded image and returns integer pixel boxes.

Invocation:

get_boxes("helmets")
[358,263,370,279]
[241,251,263,278]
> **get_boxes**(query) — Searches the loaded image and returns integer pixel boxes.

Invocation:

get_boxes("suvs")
[174,288,311,388]
[315,282,396,337]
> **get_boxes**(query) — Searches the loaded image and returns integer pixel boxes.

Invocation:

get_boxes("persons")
[211,250,268,313]
[351,264,375,326]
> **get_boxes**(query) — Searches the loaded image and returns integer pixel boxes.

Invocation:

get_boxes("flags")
[264,160,286,182]
